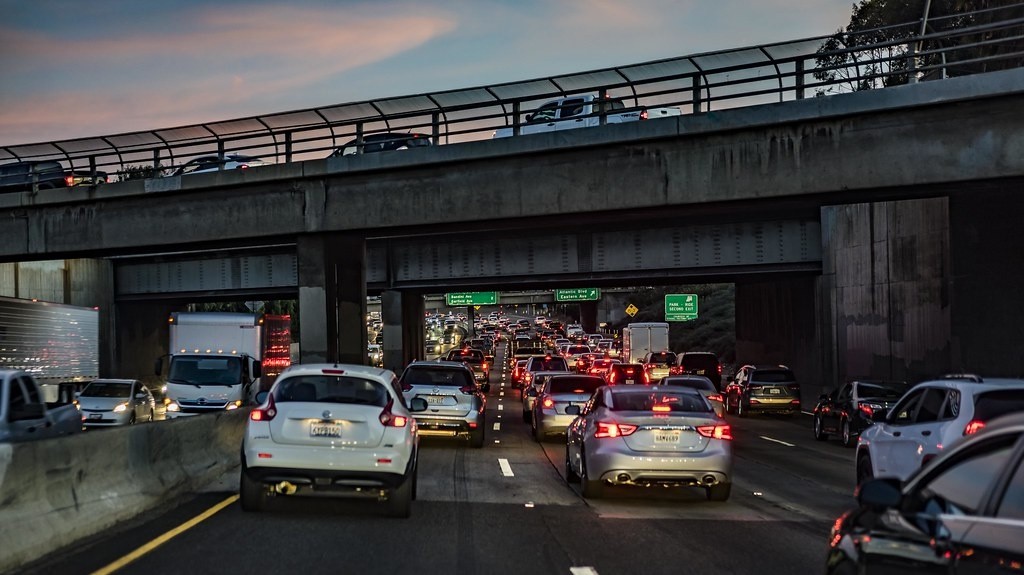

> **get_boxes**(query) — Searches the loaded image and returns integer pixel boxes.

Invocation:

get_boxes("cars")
[366,312,510,369]
[525,375,608,441]
[326,133,436,152]
[506,316,623,422]
[165,155,272,177]
[439,348,490,392]
[637,351,677,381]
[71,378,155,427]
[603,363,650,387]
[239,363,420,520]
[564,385,733,502]
[823,413,1023,575]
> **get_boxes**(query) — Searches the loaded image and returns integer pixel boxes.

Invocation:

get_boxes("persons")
[219,359,238,376]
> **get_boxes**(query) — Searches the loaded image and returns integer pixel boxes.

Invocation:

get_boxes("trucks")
[154,312,291,420]
[0,294,100,386]
[622,322,669,364]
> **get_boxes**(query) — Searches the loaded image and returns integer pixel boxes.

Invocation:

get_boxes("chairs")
[412,370,432,384]
[453,353,461,361]
[620,395,636,410]
[183,363,199,379]
[473,353,479,360]
[552,362,561,370]
[291,382,317,402]
[354,389,379,406]
[452,371,469,386]
[532,362,543,370]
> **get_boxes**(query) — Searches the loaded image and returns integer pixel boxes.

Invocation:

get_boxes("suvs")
[669,351,722,392]
[813,374,1024,485]
[397,358,487,448]
[725,364,801,418]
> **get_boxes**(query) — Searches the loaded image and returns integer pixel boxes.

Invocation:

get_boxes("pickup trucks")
[0,369,82,443]
[493,95,682,140]
[0,160,109,193]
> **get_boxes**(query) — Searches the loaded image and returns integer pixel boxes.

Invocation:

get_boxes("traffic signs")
[665,294,698,322]
[557,287,598,301]
